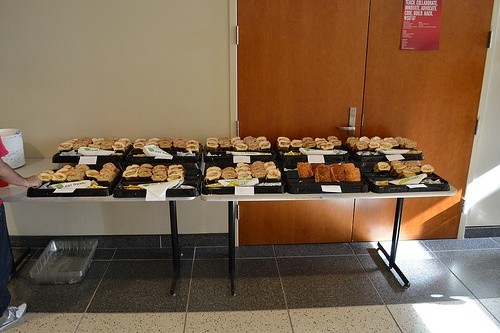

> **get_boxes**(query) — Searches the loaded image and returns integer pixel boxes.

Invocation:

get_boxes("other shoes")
[0,303,27,331]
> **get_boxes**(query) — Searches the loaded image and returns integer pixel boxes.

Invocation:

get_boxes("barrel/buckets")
[0,128,26,170]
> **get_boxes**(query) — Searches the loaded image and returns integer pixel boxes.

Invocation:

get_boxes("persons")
[0,137,42,332]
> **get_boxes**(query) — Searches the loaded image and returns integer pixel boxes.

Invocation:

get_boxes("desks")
[0,149,458,299]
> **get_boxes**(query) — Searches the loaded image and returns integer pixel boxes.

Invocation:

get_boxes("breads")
[206,136,435,181]
[37,137,199,181]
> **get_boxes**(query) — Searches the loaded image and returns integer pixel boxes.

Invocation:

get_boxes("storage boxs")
[28,240,98,284]
